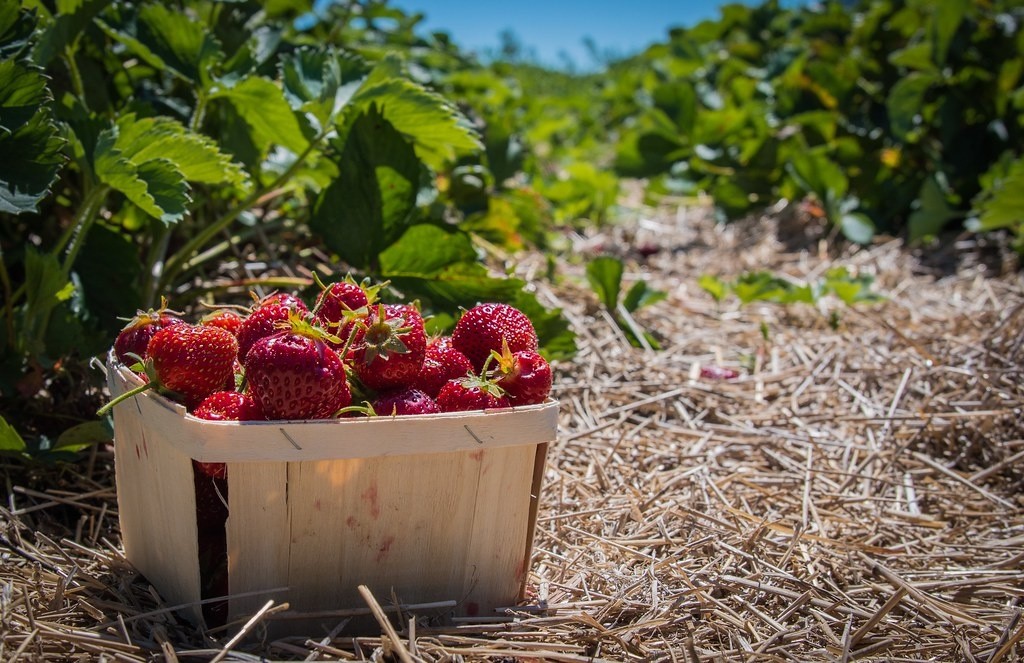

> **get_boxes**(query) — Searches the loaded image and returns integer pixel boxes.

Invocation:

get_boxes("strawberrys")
[114,270,553,637]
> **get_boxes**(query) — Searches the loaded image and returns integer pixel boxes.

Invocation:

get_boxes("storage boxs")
[105,346,562,645]
